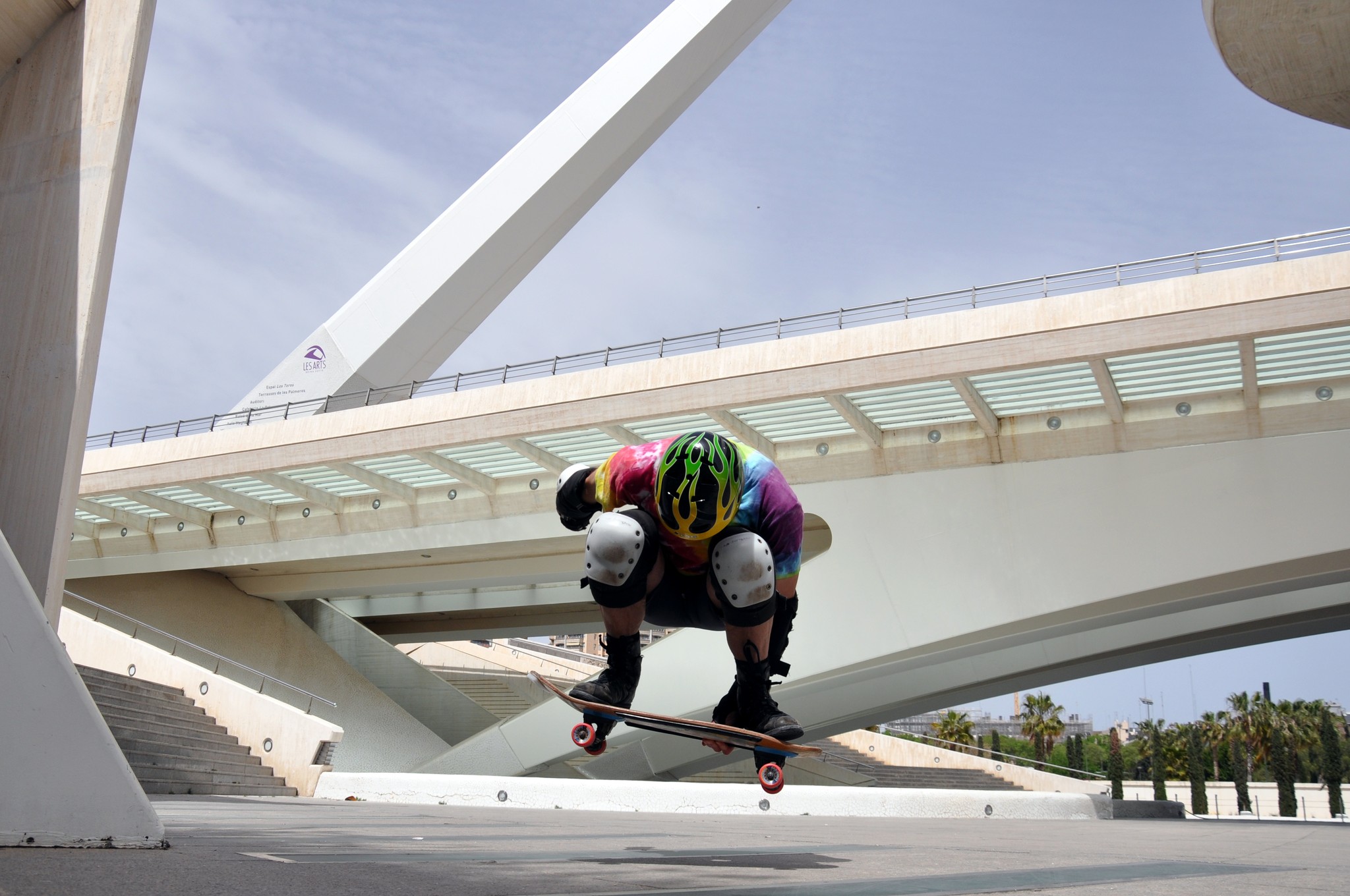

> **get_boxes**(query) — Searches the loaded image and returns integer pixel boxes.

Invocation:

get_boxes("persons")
[556,431,803,754]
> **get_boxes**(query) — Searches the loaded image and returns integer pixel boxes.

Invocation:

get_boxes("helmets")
[654,430,744,541]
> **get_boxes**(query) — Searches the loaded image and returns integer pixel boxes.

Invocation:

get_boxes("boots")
[712,639,803,741]
[569,631,643,709]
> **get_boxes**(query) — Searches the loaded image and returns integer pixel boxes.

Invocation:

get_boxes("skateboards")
[529,670,823,794]
